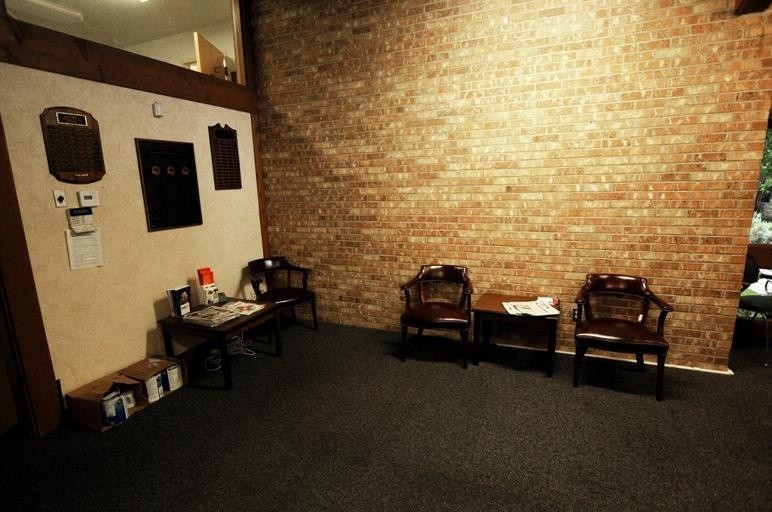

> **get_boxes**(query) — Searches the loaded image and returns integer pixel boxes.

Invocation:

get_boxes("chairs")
[247,254,319,344]
[739,254,771,367]
[395,263,472,367]
[569,273,674,400]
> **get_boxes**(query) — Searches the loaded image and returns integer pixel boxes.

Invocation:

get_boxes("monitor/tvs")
[225,56,237,82]
[193,32,225,80]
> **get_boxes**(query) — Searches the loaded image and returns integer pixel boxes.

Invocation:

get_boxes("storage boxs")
[66,355,189,435]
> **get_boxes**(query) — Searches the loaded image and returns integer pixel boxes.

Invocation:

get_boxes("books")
[166,268,265,328]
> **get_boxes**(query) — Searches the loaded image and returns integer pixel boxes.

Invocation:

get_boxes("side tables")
[158,296,282,392]
[471,292,560,379]
